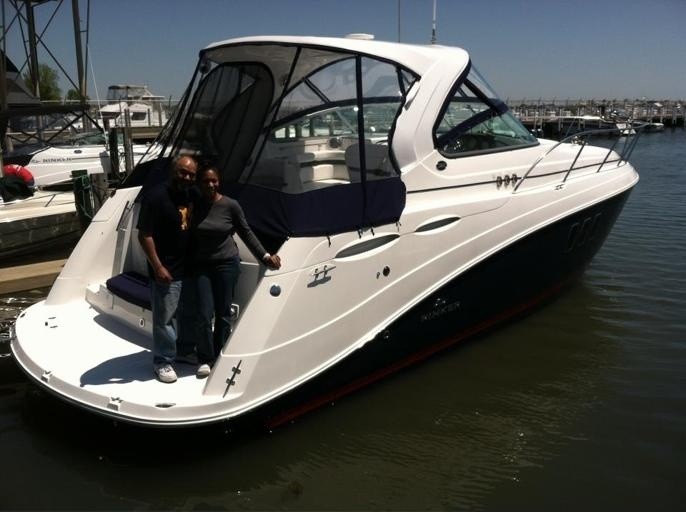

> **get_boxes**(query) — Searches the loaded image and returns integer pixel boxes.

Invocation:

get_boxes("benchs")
[261,146,348,187]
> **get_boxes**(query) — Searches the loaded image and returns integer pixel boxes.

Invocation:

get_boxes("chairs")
[341,141,393,185]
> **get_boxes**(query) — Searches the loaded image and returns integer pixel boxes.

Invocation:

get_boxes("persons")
[3,164,35,186]
[136,154,201,383]
[183,167,282,377]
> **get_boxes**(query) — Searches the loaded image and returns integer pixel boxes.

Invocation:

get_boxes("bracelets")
[263,253,270,260]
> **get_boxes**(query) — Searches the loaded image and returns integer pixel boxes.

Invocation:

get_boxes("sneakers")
[195,363,212,376]
[153,362,177,383]
[175,350,198,364]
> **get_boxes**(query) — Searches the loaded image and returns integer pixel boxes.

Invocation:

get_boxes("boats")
[275,99,686,139]
[53,83,177,130]
[8,1,650,454]
[0,165,115,259]
